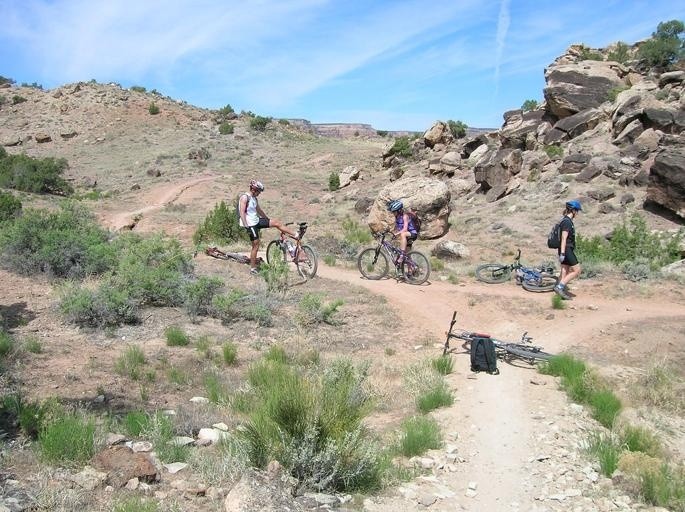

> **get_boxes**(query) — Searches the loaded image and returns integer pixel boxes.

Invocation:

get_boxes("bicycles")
[474,247,560,293]
[357,222,433,286]
[193,233,261,266]
[264,223,318,280]
[441,310,560,364]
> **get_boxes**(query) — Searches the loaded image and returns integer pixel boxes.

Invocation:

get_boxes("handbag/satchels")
[236,193,249,219]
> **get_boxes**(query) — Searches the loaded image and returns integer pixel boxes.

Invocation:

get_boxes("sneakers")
[407,265,419,277]
[554,285,571,300]
[250,268,260,275]
[398,253,406,263]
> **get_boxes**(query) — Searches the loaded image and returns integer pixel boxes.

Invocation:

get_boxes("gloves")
[559,253,566,263]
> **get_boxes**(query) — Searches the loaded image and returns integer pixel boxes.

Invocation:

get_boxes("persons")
[552,201,582,300]
[238,180,304,275]
[387,200,418,278]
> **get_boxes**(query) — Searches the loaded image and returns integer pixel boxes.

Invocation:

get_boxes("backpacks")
[548,216,572,248]
[470,337,500,375]
[402,211,421,233]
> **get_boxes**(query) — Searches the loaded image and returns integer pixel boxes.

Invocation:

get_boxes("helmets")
[249,179,265,194]
[566,201,583,211]
[386,200,403,212]
